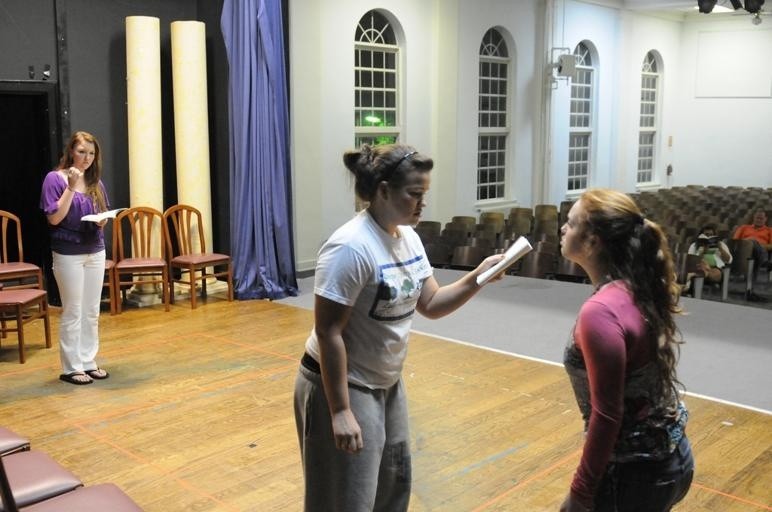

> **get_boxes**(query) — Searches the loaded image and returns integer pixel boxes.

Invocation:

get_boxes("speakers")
[556,54,576,77]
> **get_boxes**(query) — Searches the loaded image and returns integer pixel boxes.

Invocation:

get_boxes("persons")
[683,225,734,292]
[732,207,772,301]
[42,129,113,384]
[556,186,696,512]
[290,145,508,512]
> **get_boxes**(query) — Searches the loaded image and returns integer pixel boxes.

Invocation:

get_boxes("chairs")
[0,203,234,363]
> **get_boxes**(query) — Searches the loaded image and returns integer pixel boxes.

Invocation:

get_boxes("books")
[474,233,533,288]
[81,204,130,225]
[696,234,721,248]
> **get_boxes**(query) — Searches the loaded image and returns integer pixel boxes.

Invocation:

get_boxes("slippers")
[85,367,108,379]
[60,372,93,385]
